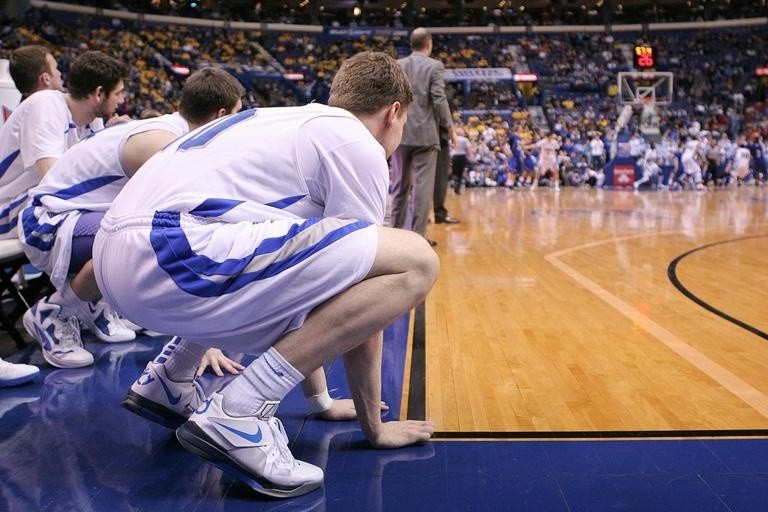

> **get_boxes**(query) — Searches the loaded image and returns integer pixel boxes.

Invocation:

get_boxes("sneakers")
[121,361,325,499]
[0,295,136,387]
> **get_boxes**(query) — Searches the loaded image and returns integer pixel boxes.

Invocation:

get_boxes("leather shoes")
[428,240,437,246]
[435,214,460,224]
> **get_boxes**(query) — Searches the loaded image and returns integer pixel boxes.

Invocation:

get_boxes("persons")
[384,25,457,246]
[21,67,244,375]
[92,51,440,499]
[434,125,460,224]
[1,49,125,244]
